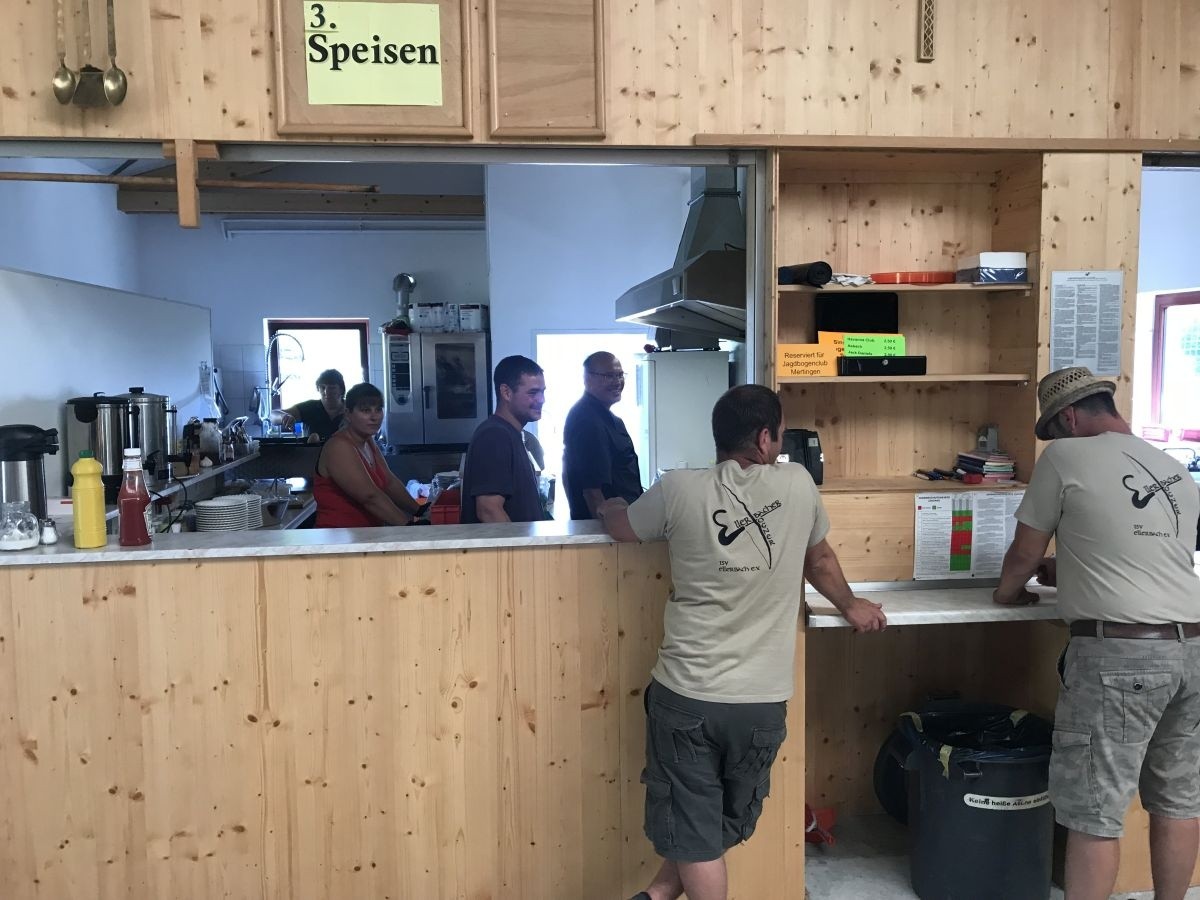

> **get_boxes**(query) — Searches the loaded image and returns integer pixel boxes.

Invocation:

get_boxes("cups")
[295,422,303,436]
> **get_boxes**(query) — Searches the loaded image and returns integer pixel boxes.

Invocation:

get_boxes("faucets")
[266,332,305,429]
[142,448,161,475]
[176,437,194,467]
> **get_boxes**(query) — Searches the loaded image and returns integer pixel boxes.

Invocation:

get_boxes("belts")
[1073,619,1200,639]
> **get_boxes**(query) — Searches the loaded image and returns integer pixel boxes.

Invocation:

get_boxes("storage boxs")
[428,491,461,525]
[955,252,1028,283]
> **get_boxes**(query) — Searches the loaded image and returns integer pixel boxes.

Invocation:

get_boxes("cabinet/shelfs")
[763,149,1047,498]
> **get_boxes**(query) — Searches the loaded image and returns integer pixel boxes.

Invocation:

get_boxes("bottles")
[71,449,108,549]
[223,440,234,461]
[200,418,223,464]
[1,501,41,550]
[39,519,59,546]
[117,448,156,544]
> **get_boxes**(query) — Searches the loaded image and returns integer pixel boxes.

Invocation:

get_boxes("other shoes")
[632,891,650,900]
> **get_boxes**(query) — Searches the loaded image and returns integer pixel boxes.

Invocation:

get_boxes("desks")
[804,586,1062,627]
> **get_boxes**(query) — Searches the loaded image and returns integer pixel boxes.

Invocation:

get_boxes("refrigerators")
[635,350,730,494]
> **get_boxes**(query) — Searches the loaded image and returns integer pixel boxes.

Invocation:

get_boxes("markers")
[914,468,954,480]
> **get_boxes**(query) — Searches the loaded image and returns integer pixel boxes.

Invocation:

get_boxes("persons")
[994,365,1200,900]
[597,385,888,900]
[270,369,346,439]
[312,383,430,529]
[459,355,555,524]
[561,350,644,520]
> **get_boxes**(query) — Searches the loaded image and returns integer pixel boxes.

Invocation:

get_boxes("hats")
[1035,366,1117,441]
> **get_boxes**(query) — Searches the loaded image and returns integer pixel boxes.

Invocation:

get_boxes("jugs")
[1,423,60,526]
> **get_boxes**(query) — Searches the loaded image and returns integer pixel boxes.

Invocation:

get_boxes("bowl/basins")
[261,497,289,526]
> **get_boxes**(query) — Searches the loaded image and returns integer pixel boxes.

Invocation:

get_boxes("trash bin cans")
[903,704,1056,900]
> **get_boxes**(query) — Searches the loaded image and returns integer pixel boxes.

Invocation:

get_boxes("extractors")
[614,164,747,344]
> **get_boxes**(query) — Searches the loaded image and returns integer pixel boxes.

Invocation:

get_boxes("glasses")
[587,371,625,380]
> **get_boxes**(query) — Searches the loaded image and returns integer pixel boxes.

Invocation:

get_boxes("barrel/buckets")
[64,392,160,504]
[114,387,193,487]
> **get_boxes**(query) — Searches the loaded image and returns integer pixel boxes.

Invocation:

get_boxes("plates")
[193,494,265,532]
